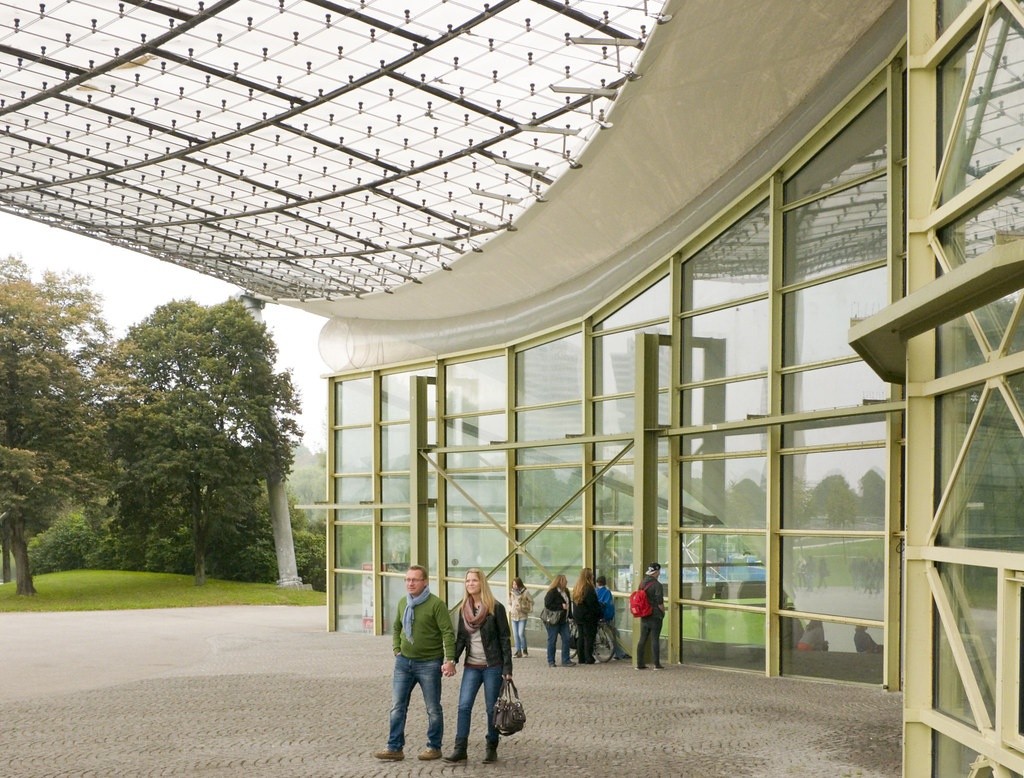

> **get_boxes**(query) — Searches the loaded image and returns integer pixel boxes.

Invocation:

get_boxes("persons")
[572,568,607,665]
[594,576,616,626]
[796,620,829,652]
[441,568,513,764]
[853,625,883,653]
[796,553,883,597]
[373,565,457,761]
[634,563,667,671]
[541,575,577,668]
[508,577,535,659]
[785,607,804,648]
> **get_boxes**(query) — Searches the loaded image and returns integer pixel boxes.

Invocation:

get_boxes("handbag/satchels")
[539,607,564,625]
[493,679,526,736]
[569,636,577,649]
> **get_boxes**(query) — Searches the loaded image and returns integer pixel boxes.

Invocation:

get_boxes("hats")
[645,563,661,575]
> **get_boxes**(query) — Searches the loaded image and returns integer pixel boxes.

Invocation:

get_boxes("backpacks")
[630,580,657,617]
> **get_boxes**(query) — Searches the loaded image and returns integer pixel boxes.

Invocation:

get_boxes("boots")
[482,734,499,764]
[442,737,468,765]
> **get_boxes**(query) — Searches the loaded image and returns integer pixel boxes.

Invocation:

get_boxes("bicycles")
[569,619,618,663]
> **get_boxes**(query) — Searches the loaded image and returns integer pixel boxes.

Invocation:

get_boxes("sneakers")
[654,664,666,670]
[636,667,649,670]
[374,748,405,759]
[417,748,442,760]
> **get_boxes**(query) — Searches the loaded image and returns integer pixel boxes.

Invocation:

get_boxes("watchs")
[445,660,456,666]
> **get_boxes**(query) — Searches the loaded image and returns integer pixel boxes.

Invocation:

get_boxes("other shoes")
[513,652,522,658]
[595,659,601,664]
[549,663,557,667]
[562,662,576,667]
[523,650,528,658]
[614,654,632,660]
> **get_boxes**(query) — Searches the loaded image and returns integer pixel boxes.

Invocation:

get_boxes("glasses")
[404,579,424,583]
